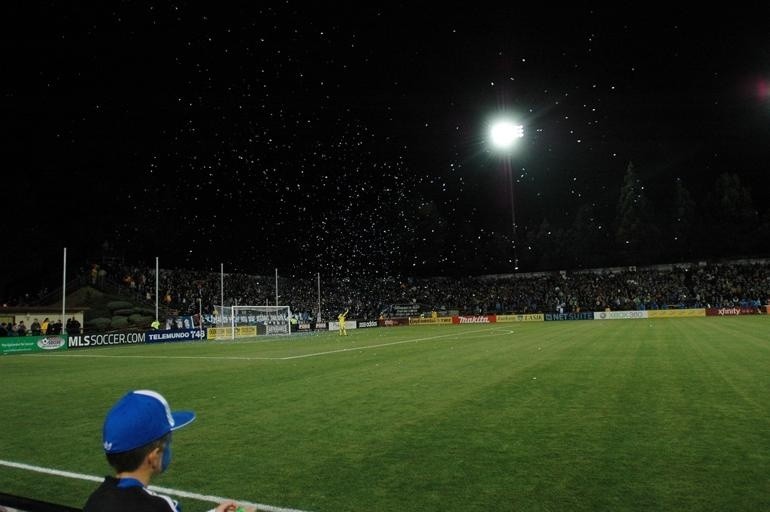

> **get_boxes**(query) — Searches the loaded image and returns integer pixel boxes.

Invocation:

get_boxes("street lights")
[488,122,524,274]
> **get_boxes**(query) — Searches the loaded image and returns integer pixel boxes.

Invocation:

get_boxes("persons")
[338,308,352,338]
[98,264,769,331]
[0,316,82,336]
[84,390,256,512]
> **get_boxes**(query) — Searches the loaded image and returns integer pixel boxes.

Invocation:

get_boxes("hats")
[102,387,195,454]
